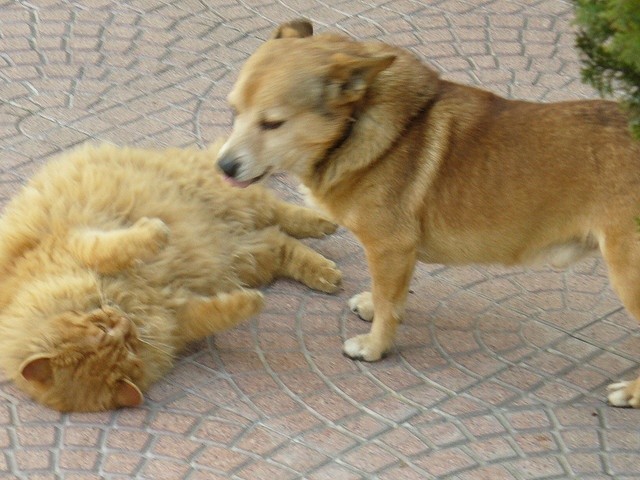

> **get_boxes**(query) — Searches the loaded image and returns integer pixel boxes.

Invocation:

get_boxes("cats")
[1,139,348,416]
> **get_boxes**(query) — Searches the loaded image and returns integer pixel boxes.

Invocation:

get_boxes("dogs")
[213,15,639,412]
